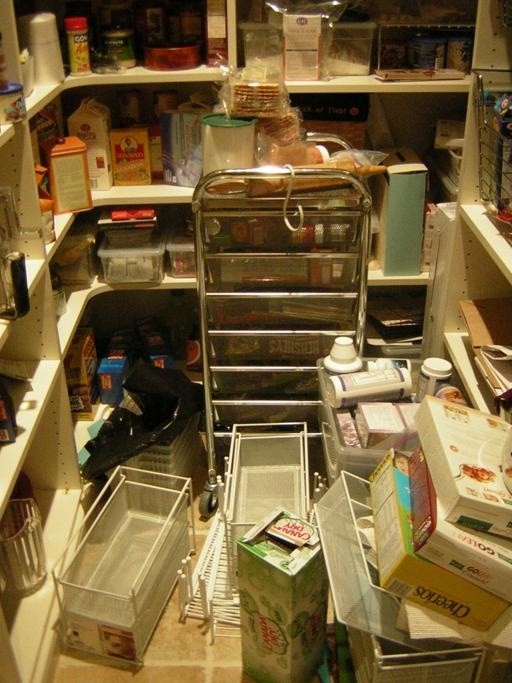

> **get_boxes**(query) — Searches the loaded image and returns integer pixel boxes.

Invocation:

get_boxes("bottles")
[64,17,91,75]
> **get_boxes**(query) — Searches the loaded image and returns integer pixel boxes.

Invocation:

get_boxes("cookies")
[229,83,299,146]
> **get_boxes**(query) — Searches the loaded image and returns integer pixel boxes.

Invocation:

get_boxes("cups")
[199,113,259,196]
[42,211,57,243]
[51,289,67,318]
[2,244,31,321]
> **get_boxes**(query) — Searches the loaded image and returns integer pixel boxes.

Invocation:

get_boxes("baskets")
[475,74,511,244]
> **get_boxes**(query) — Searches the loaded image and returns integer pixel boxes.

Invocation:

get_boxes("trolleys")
[192,165,372,519]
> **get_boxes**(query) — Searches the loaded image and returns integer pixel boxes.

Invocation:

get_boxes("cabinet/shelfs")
[0,0,509,681]
